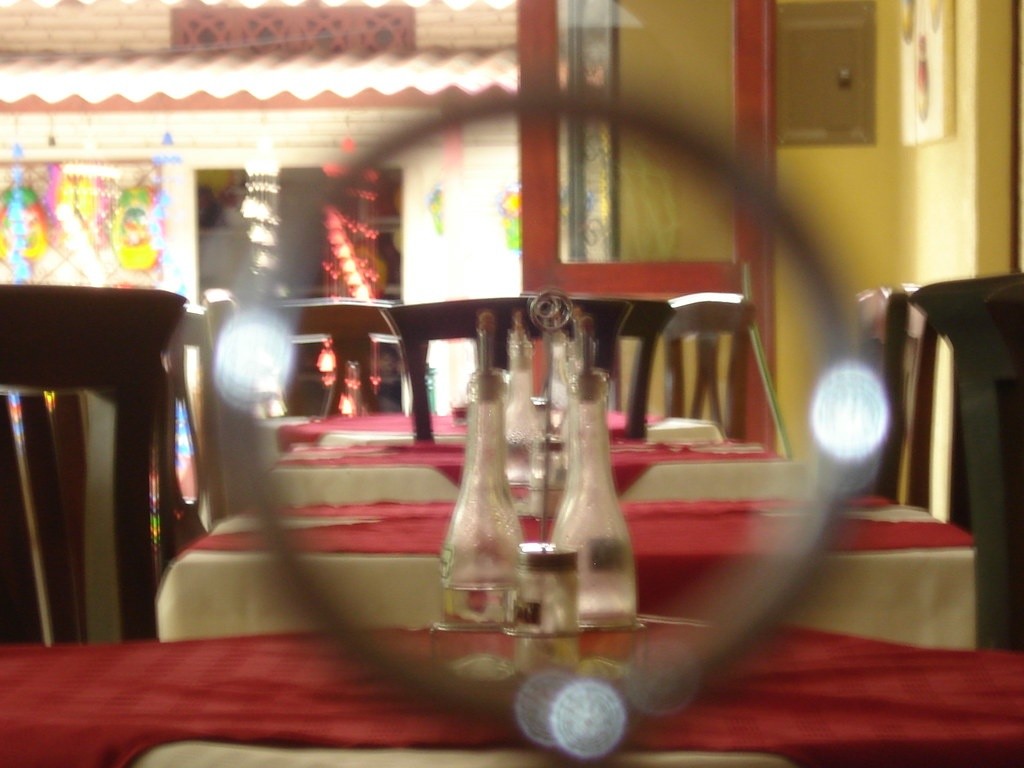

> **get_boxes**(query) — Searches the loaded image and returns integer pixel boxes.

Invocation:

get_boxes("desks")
[152,501,979,651]
[261,411,724,452]
[268,443,796,503]
[0,612,1024,768]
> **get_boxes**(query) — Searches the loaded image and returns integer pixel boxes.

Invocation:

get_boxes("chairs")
[851,281,942,527]
[178,285,758,532]
[0,282,188,642]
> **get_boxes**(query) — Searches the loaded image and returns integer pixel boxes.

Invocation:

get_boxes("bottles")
[510,539,580,683]
[440,306,635,624]
